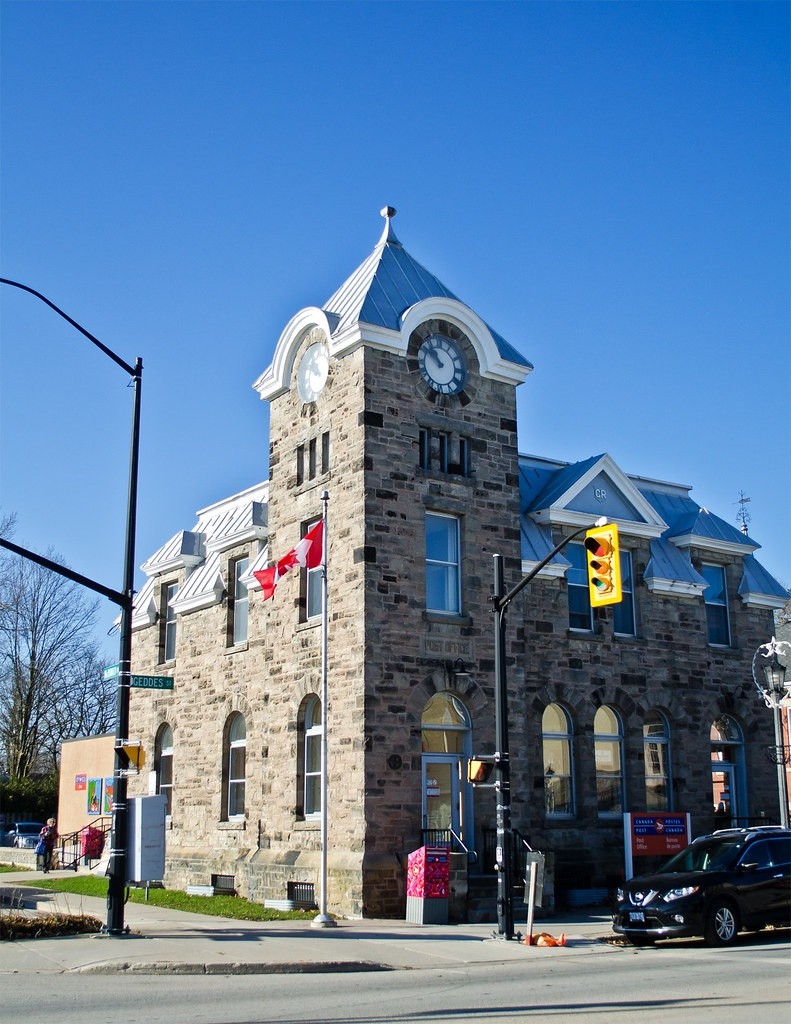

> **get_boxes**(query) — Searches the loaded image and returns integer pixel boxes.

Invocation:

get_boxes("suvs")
[610,824,791,946]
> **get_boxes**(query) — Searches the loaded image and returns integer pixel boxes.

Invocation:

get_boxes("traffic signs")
[101,663,120,682]
[129,675,174,690]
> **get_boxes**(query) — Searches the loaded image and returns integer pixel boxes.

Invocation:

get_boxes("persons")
[33,817,60,873]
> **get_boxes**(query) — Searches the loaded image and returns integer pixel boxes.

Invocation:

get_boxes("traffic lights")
[584,524,623,608]
[115,744,146,772]
[468,759,494,784]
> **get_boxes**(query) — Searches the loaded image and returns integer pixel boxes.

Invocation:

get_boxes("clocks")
[296,341,330,404]
[417,334,467,396]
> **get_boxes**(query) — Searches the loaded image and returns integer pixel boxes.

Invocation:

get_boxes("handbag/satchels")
[33,833,47,856]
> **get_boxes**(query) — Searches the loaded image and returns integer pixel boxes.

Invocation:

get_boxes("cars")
[0,821,47,849]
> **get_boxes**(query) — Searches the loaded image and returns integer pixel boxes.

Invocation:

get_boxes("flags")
[254,517,325,603]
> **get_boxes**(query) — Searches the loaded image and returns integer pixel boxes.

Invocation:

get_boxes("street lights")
[760,637,789,831]
